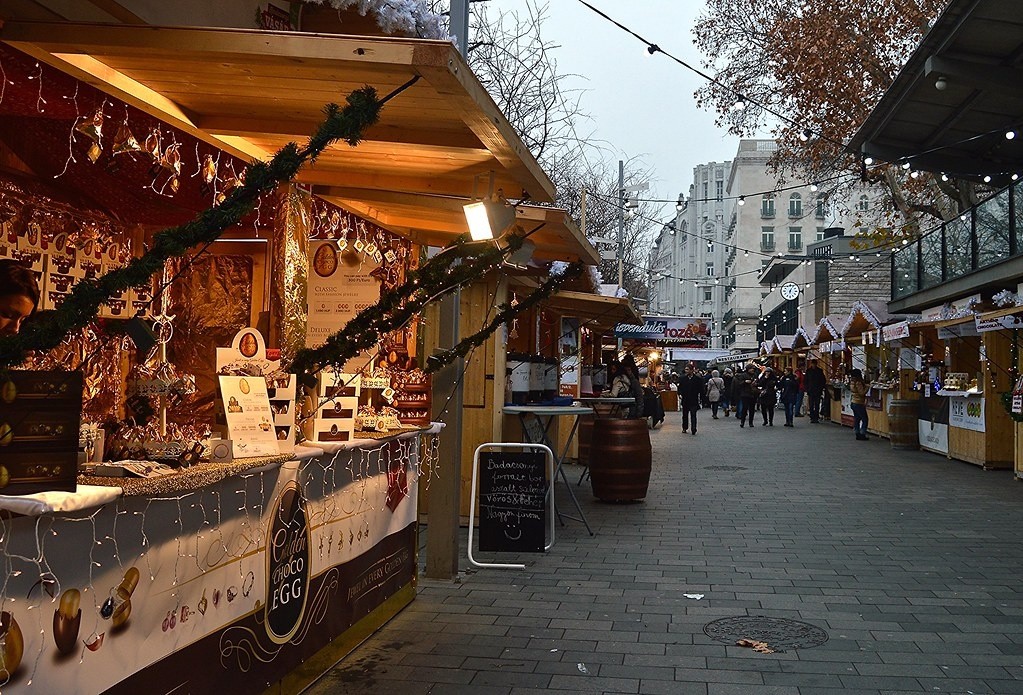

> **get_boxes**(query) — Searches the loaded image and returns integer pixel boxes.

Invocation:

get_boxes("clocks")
[780,282,804,301]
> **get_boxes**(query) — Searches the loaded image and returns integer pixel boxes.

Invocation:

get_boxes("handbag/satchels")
[615,381,633,406]
[720,389,726,401]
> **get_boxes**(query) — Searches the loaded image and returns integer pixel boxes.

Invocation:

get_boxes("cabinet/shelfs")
[373,354,432,425]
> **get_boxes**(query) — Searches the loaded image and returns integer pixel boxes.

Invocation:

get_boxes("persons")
[848,368,870,441]
[600,355,832,436]
[0,258,40,340]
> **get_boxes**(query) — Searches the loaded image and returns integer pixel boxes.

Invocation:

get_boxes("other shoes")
[811,418,819,423]
[784,422,789,426]
[795,414,804,417]
[682,428,687,433]
[725,410,729,417]
[740,422,744,427]
[749,422,754,427]
[763,421,768,426]
[692,429,695,435]
[856,433,870,440]
[769,419,773,426]
[790,422,794,427]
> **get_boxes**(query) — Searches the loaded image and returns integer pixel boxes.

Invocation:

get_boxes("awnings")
[839,0,1023,185]
[20,22,645,326]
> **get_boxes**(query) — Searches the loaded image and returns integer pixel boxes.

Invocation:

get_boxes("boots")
[712,411,719,419]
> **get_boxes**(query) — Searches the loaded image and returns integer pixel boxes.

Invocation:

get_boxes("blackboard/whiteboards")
[479,451,547,552]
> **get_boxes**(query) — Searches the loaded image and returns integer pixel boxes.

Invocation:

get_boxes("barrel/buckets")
[887,399,919,450]
[507,348,559,404]
[590,418,652,499]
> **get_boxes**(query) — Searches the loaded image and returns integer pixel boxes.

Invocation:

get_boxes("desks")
[573,397,635,487]
[502,405,595,538]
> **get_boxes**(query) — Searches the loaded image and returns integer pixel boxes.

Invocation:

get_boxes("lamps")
[496,224,536,270]
[463,170,515,242]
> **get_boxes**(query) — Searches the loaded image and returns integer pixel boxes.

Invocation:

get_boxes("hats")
[765,367,773,372]
